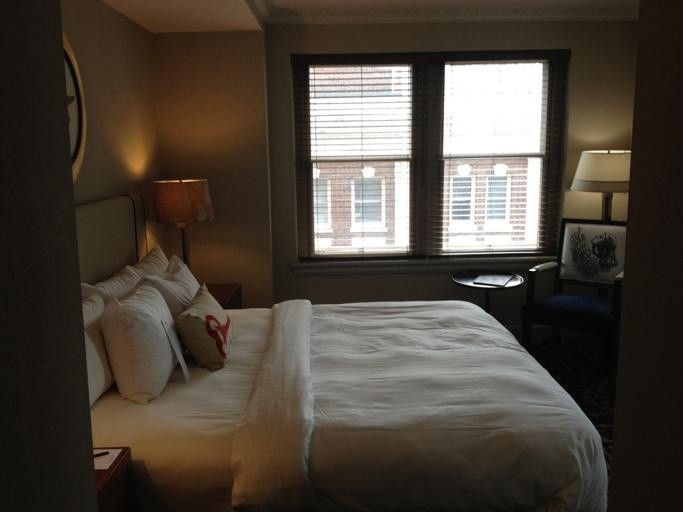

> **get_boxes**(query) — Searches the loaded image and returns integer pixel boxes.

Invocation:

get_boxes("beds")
[72,189,608,511]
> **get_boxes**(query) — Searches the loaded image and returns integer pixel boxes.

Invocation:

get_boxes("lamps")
[149,174,216,269]
[569,147,633,222]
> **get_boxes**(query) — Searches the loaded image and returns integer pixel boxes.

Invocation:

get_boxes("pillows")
[75,239,234,410]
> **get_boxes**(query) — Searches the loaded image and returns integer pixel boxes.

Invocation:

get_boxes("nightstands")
[200,281,242,310]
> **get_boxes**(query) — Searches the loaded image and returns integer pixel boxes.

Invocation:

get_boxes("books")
[473,275,514,287]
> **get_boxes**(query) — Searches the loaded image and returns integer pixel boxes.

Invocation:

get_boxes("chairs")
[520,217,628,362]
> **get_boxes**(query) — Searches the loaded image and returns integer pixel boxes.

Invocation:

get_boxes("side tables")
[451,266,524,318]
